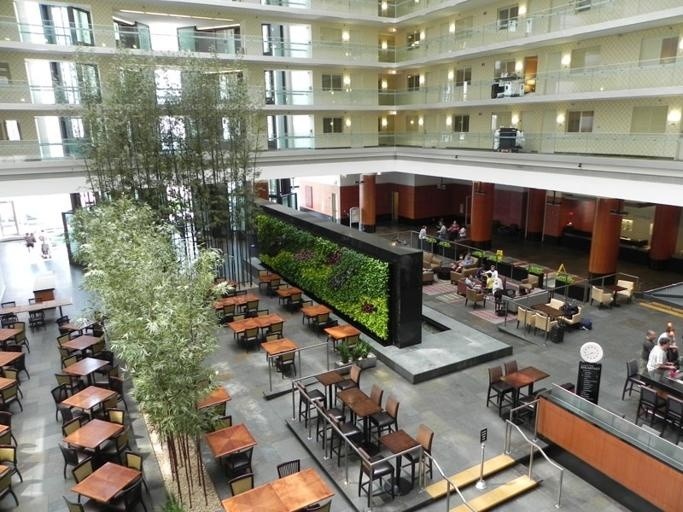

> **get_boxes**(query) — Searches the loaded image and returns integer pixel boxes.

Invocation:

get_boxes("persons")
[647,337,676,372]
[24,229,51,260]
[657,322,680,369]
[639,330,656,371]
[417,218,502,298]
